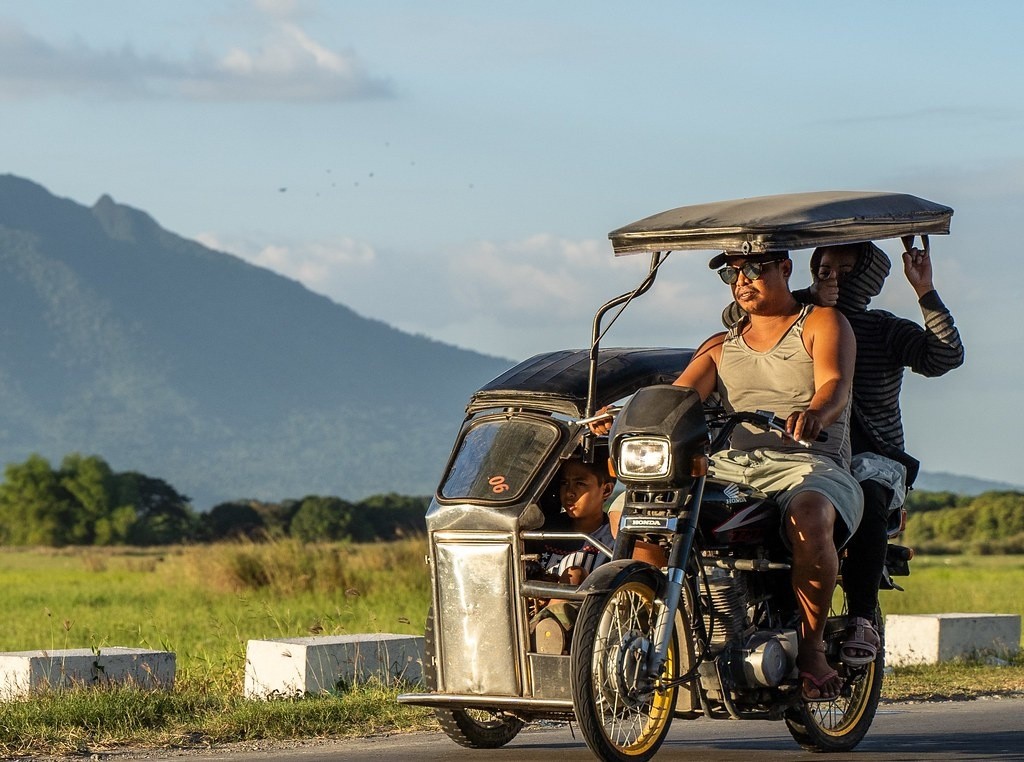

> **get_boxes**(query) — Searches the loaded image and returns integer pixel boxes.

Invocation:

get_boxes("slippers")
[797,668,841,703]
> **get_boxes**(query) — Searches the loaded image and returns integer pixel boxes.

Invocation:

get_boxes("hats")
[709,250,790,271]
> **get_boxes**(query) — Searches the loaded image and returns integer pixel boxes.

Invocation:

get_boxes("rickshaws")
[398,189,955,762]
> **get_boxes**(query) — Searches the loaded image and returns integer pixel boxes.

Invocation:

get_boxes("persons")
[525,450,618,655]
[589,252,863,702]
[791,243,965,666]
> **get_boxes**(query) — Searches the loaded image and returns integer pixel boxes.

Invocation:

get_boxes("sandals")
[840,616,881,666]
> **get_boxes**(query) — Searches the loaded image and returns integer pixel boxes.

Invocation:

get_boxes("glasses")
[717,258,785,285]
